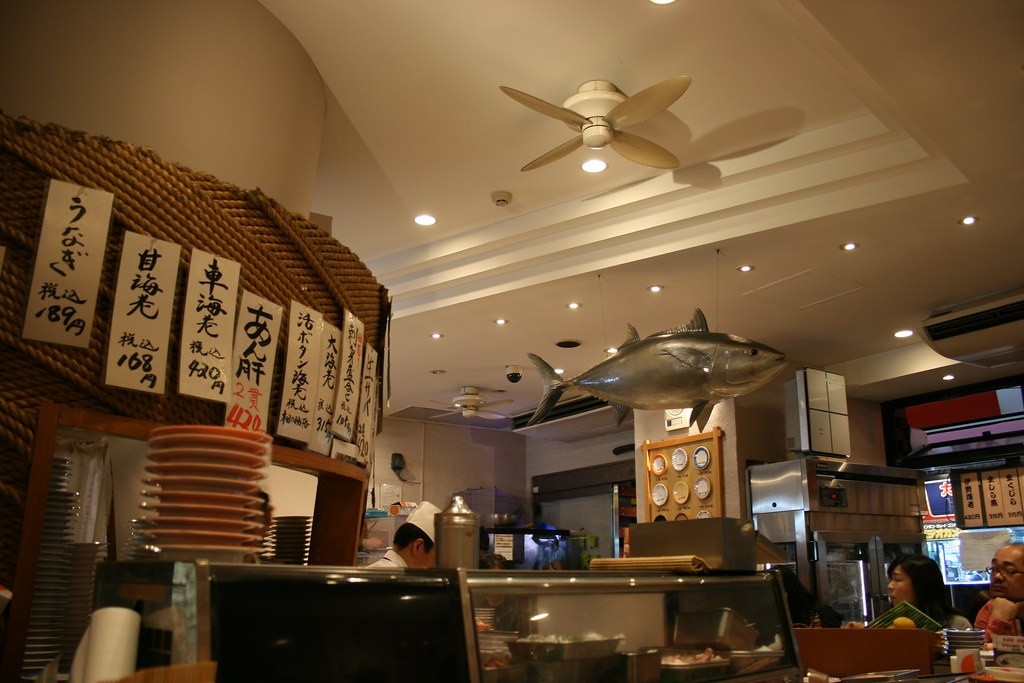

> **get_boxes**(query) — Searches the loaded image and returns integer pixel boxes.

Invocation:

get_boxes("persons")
[469,554,532,661]
[366,502,441,568]
[887,554,973,631]
[974,542,1024,643]
[221,492,292,583]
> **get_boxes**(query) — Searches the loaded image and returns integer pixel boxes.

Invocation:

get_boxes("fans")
[500,73,693,173]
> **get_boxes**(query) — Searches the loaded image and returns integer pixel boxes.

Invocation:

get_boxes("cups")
[950,656,956,673]
[955,649,980,672]
[86,607,142,683]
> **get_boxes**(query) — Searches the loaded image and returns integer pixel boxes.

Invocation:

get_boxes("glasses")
[986,567,1024,578]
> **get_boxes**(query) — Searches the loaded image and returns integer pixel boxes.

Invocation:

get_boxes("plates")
[122,423,311,566]
[674,481,689,503]
[697,510,711,518]
[652,454,667,475]
[654,515,666,523]
[472,606,498,632]
[21,457,109,672]
[672,448,687,471]
[694,446,710,469]
[675,513,687,521]
[694,476,710,499]
[943,626,986,660]
[652,484,668,506]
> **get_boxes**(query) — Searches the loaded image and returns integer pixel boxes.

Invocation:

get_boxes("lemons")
[893,616,917,629]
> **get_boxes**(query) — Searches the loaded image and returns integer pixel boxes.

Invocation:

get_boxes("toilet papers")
[68,607,141,683]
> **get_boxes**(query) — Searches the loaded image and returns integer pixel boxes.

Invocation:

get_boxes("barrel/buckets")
[434,512,481,570]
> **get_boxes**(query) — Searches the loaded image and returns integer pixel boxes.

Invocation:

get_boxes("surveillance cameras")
[504,365,522,384]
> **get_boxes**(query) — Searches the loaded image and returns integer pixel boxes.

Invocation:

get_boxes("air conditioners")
[915,288,1024,358]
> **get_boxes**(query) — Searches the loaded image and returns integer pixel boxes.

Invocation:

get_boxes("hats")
[405,501,442,545]
